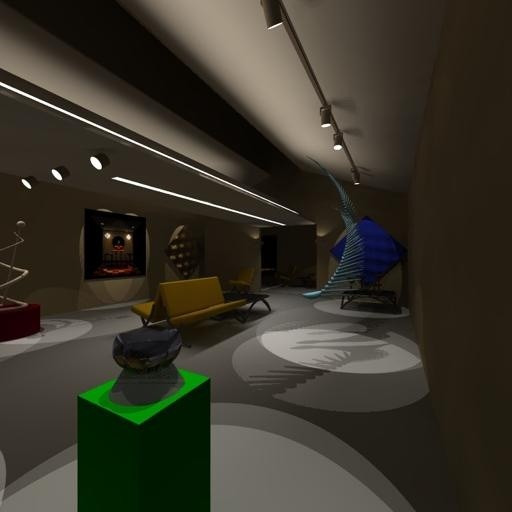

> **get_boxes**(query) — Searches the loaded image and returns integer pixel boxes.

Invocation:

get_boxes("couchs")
[160,276,250,349]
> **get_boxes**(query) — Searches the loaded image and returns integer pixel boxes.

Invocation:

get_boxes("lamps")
[18,149,111,191]
[314,102,360,186]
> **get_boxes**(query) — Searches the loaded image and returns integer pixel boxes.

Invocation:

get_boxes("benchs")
[130,282,166,328]
[222,292,270,312]
